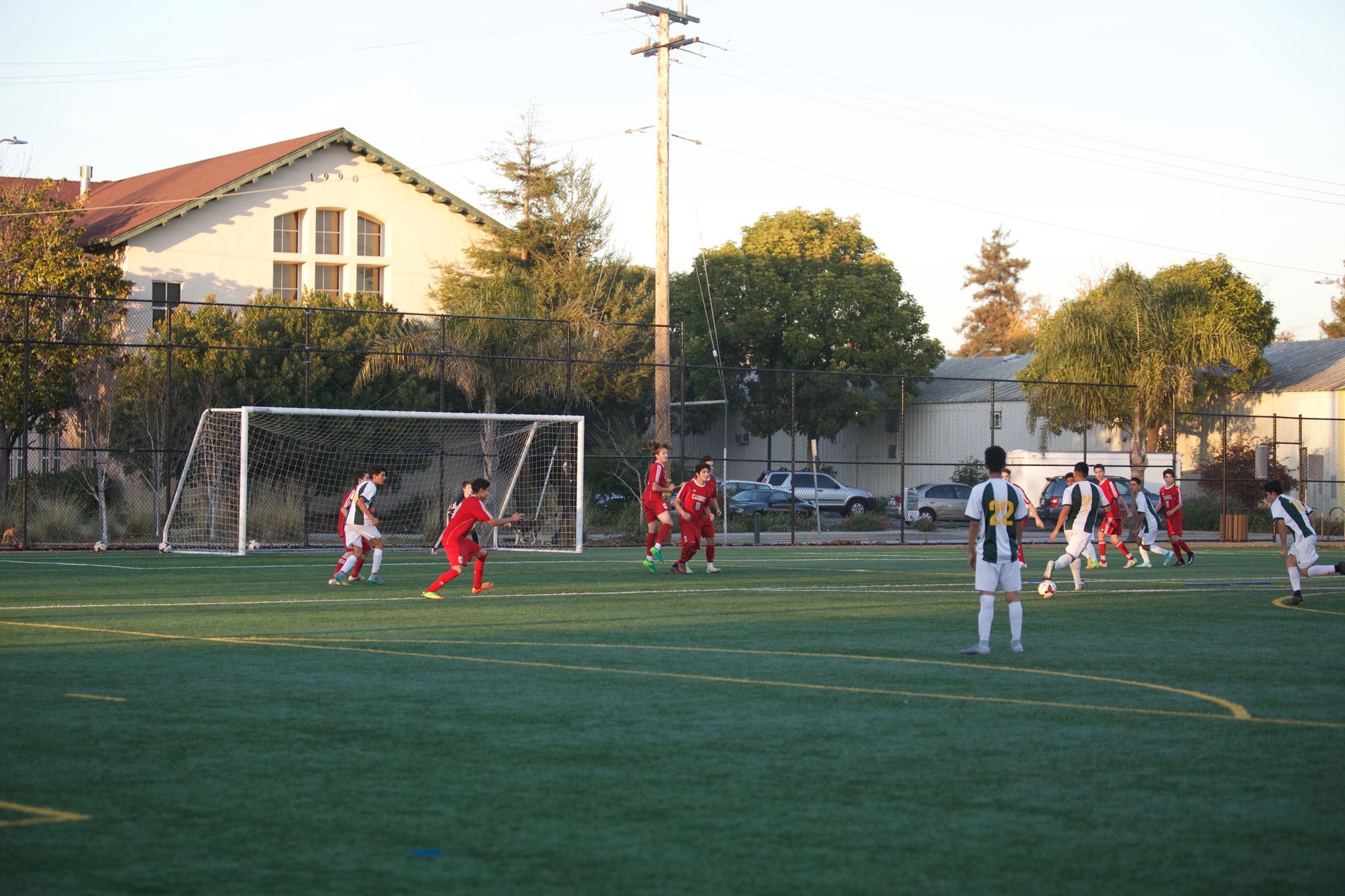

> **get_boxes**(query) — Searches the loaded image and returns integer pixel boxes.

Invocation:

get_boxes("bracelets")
[708,512,712,517]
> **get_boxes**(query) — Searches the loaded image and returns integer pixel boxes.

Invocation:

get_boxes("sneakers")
[1043,550,1195,592]
[1019,561,1027,569]
[1285,596,1303,605]
[431,548,438,555]
[1340,561,1345,575]
[685,565,693,573]
[960,643,990,655]
[328,579,341,585]
[472,581,493,595]
[334,573,352,586]
[421,591,444,599]
[678,561,687,574]
[368,575,385,584]
[670,562,682,575]
[649,545,664,563]
[707,566,722,574]
[348,574,366,582]
[1011,639,1024,654]
[643,558,659,574]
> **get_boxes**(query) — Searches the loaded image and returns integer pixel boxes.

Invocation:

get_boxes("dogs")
[2,524,20,543]
[502,515,551,546]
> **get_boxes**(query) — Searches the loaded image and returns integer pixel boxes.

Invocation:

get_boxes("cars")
[1035,474,1166,531]
[727,489,817,520]
[885,483,973,528]
[716,480,775,499]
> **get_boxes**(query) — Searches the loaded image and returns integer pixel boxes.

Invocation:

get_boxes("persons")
[960,446,1027,655]
[1000,461,1196,592]
[642,441,723,574]
[1265,480,1345,606]
[330,462,589,600]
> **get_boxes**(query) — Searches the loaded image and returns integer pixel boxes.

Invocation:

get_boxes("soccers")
[1037,581,1057,598]
[247,540,260,550]
[94,541,107,553]
[159,541,172,553]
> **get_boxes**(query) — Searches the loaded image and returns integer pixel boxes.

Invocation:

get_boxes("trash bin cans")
[1220,507,1250,542]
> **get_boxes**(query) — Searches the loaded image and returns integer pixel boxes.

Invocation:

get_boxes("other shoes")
[547,541,587,546]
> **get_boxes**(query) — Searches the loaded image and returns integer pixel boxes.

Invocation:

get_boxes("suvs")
[758,470,879,519]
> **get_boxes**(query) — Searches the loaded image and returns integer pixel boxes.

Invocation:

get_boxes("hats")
[563,462,572,467]
